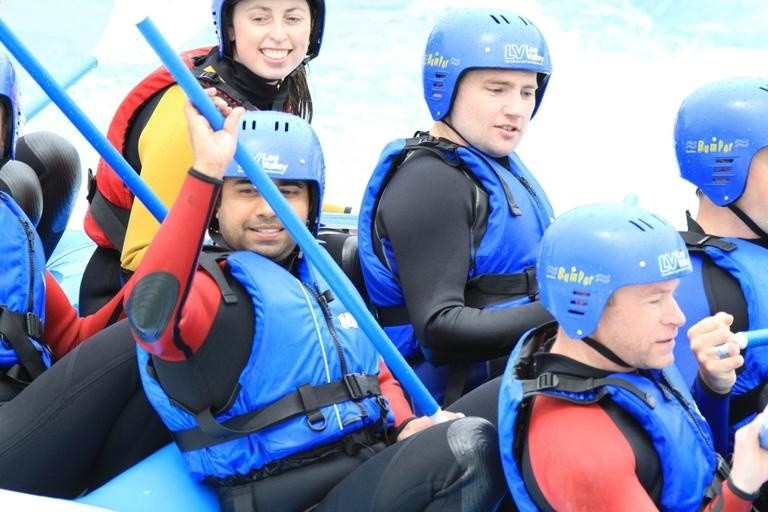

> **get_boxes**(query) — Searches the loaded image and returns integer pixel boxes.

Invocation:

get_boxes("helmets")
[537,204,692,339]
[0,52,20,161]
[423,9,552,121]
[675,79,768,206]
[212,112,325,238]
[212,1,326,58]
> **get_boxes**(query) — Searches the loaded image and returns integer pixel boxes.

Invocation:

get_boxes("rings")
[717,345,730,359]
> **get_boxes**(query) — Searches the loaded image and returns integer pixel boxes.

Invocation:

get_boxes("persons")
[78,0,367,316]
[674,75,768,464]
[0,132,83,265]
[359,6,555,412]
[498,203,768,512]
[126,87,516,512]
[0,55,174,500]
